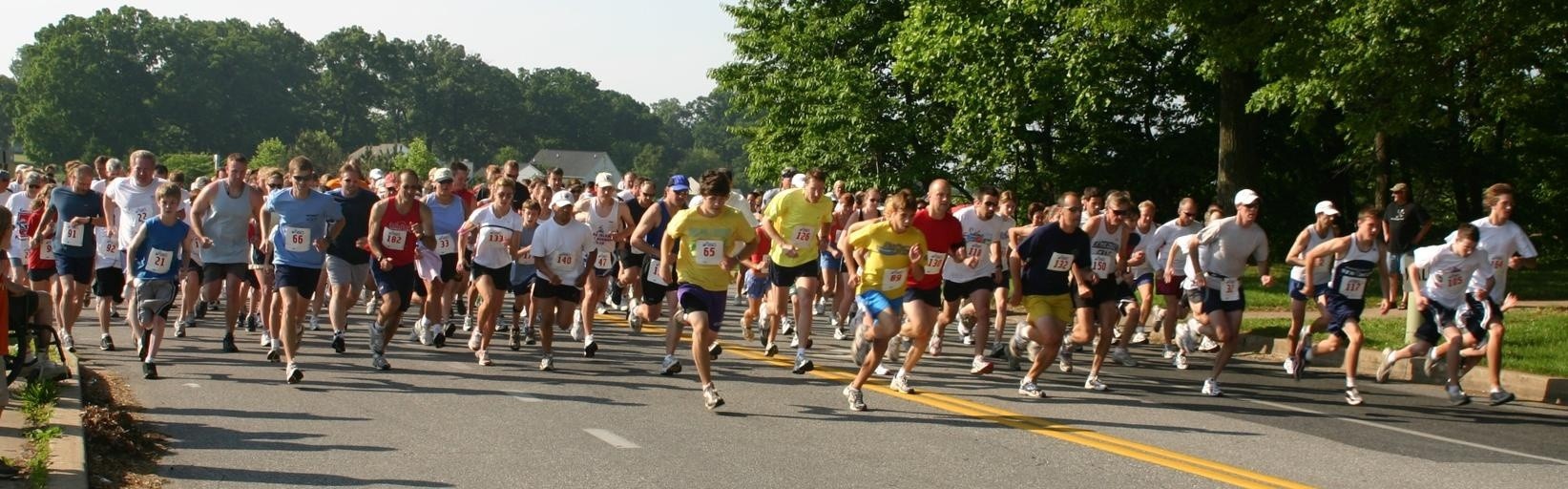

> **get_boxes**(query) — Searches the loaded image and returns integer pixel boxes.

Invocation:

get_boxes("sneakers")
[1345,386,1363,405]
[673,309,689,328]
[708,342,722,360]
[1284,325,1313,376]
[703,383,724,410]
[1424,347,1441,377]
[19,358,39,377]
[630,297,642,335]
[1490,389,1515,406]
[364,292,628,371]
[1446,390,1471,405]
[60,285,348,384]
[660,355,681,376]
[740,295,1223,411]
[39,363,71,379]
[1376,347,1393,383]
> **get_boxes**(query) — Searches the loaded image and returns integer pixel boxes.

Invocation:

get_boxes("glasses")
[1183,212,1195,217]
[1062,206,1083,213]
[267,183,283,190]
[293,175,313,181]
[984,201,998,207]
[869,198,881,202]
[1108,205,1128,215]
[642,192,654,197]
[1243,204,1260,208]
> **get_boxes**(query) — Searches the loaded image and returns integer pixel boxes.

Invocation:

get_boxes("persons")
[2,148,1540,410]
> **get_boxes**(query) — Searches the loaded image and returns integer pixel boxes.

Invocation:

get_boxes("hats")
[1315,200,1341,217]
[1390,183,1408,193]
[550,172,614,208]
[369,168,398,197]
[195,176,212,192]
[781,167,807,189]
[1,164,41,186]
[1234,189,1260,205]
[667,174,690,192]
[433,168,454,183]
